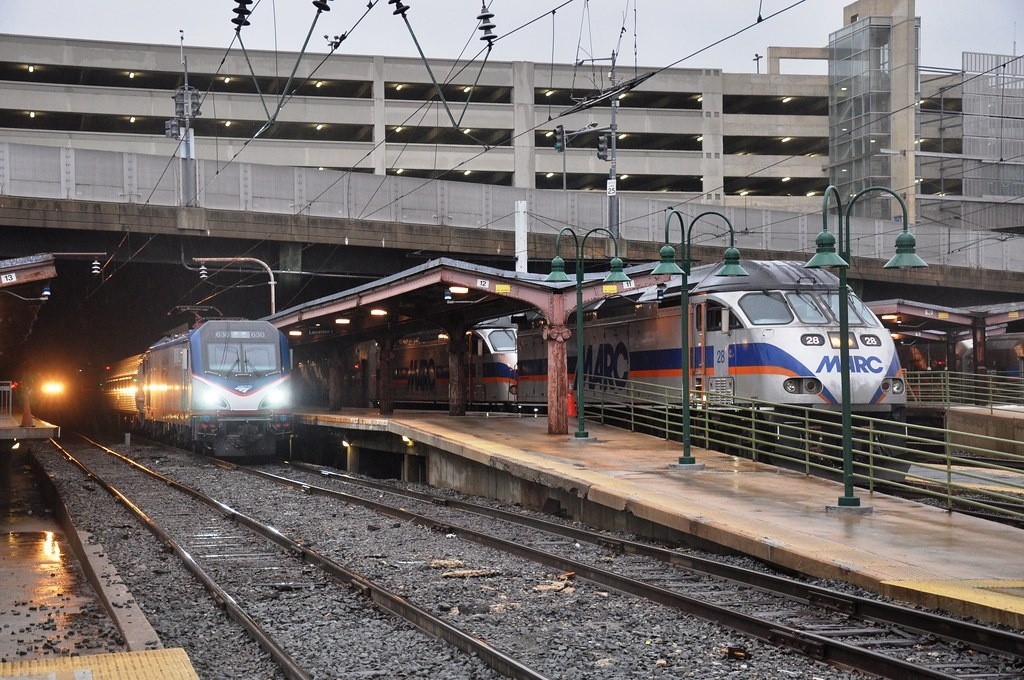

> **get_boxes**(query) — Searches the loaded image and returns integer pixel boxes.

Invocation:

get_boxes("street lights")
[100,320,297,459]
[650,210,750,465]
[804,185,928,506]
[544,227,632,437]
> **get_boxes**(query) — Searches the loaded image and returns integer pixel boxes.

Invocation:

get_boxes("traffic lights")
[553,124,564,152]
[597,136,608,160]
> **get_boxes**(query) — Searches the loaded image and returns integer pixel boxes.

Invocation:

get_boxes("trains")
[508,258,914,479]
[294,324,517,410]
